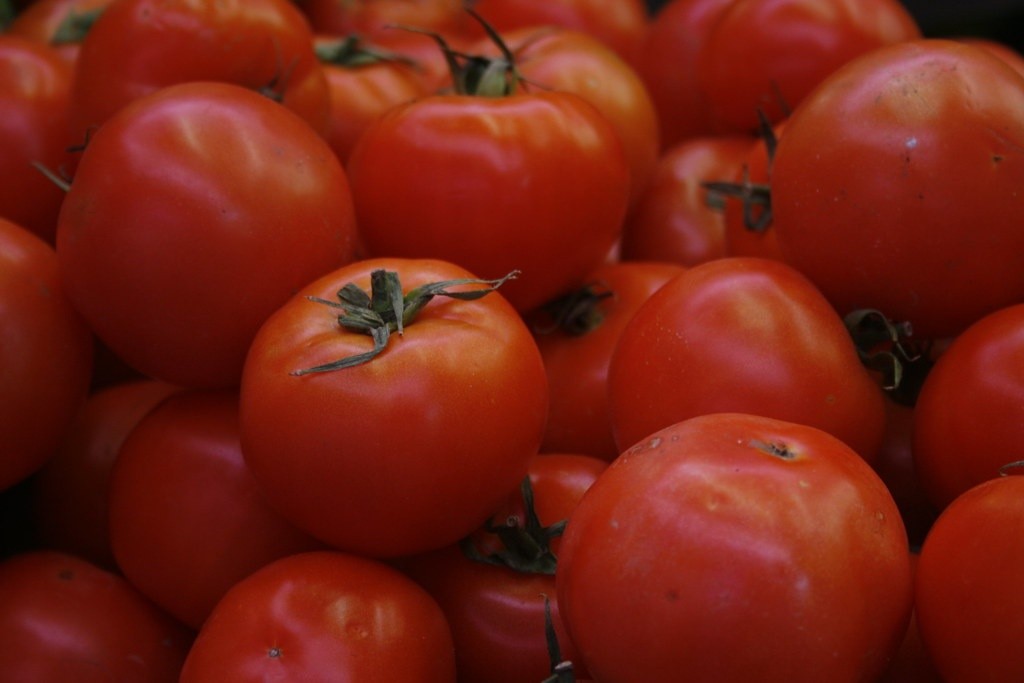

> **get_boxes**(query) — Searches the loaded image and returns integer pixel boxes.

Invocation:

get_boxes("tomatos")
[0,1,1024,683]
